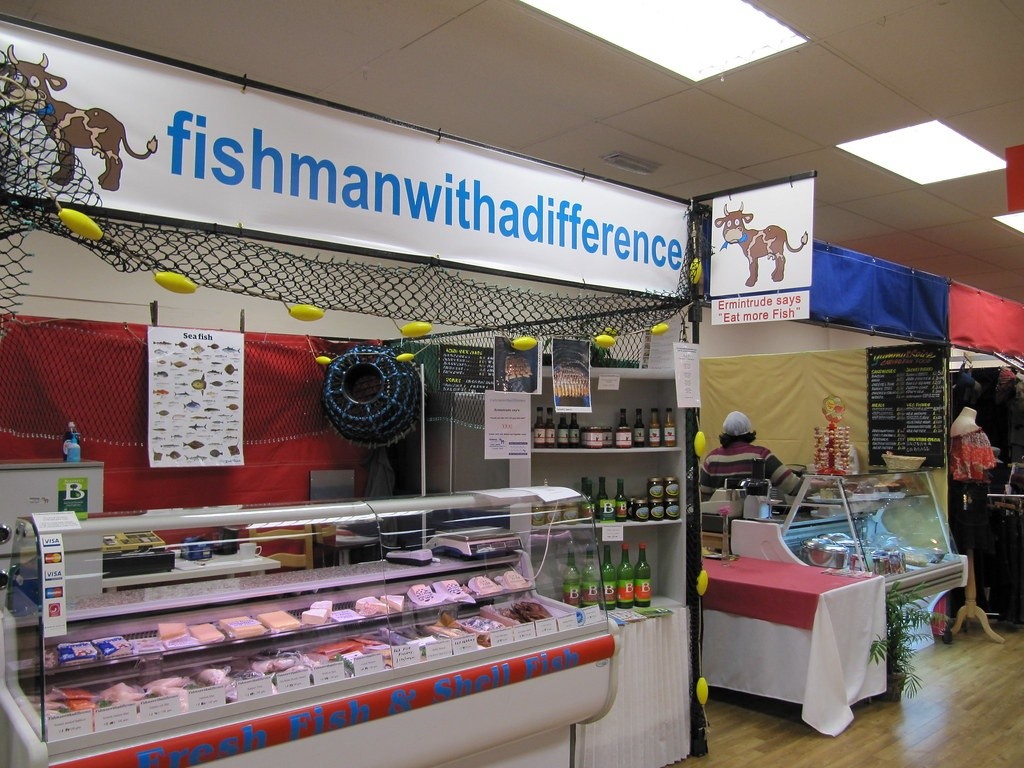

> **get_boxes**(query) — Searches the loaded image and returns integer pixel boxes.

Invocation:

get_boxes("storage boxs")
[179,537,212,561]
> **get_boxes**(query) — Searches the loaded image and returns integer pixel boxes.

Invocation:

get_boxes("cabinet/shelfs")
[0,486,622,768]
[509,366,690,614]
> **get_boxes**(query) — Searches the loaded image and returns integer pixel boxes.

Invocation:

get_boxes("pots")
[816,533,865,564]
[799,538,848,569]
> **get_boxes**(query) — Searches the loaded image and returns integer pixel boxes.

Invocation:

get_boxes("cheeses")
[301,594,405,625]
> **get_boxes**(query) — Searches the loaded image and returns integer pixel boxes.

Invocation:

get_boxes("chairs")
[248,524,313,574]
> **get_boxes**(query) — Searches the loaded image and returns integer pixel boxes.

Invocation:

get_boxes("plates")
[806,494,906,502]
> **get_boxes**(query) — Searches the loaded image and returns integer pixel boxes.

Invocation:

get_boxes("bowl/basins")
[820,484,901,499]
[898,546,949,564]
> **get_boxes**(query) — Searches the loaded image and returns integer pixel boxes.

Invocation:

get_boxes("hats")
[723,411,752,436]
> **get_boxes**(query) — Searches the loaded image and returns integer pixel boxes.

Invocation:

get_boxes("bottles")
[63,421,80,462]
[579,476,588,519]
[616,543,634,609]
[580,546,599,607]
[599,544,616,610]
[545,407,556,449]
[649,408,660,446]
[663,408,675,447]
[633,408,645,447]
[615,478,627,522]
[634,543,651,608]
[569,412,580,449]
[534,406,545,449]
[583,480,595,523]
[556,413,569,449]
[618,408,628,427]
[594,477,608,519]
[563,547,579,609]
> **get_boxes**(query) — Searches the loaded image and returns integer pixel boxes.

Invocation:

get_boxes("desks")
[315,534,380,571]
[702,549,887,737]
[100,550,281,593]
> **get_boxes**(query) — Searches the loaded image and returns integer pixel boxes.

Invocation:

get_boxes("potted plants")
[869,580,951,701]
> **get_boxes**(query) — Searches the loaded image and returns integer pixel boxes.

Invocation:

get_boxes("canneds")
[532,501,579,526]
[850,549,907,575]
[626,476,679,522]
[580,425,632,449]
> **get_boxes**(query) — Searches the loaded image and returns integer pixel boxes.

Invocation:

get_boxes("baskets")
[881,454,926,473]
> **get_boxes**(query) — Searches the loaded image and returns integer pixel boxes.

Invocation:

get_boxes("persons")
[949,407,996,555]
[699,410,803,502]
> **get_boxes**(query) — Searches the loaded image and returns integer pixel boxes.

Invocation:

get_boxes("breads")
[905,553,927,566]
